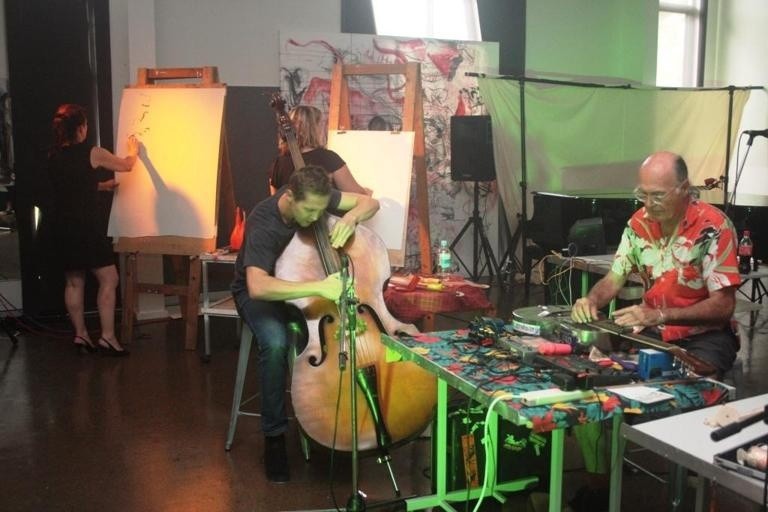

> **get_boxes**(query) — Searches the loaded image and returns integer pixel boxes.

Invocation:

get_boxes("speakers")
[450,116,496,180]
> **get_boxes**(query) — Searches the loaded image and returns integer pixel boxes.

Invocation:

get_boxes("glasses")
[632,178,689,207]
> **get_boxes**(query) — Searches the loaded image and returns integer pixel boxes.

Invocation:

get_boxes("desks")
[555,255,766,318]
[383,303,737,512]
[619,393,768,511]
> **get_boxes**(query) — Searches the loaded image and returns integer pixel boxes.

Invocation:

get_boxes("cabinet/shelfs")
[199,252,241,356]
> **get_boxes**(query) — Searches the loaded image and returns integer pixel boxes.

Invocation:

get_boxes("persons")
[46,105,138,357]
[231,166,380,485]
[269,106,373,197]
[503,152,740,512]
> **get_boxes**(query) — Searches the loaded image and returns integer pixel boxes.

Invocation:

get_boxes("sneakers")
[261,429,292,483]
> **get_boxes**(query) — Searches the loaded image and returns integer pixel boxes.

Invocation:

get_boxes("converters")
[550,373,574,390]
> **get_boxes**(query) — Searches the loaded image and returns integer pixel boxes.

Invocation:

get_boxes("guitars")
[513,305,715,375]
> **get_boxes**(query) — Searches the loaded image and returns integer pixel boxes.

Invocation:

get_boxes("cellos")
[269,90,451,457]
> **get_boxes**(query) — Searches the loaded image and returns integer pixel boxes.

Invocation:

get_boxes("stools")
[224,312,333,462]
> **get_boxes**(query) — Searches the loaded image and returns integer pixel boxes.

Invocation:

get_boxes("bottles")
[736,230,754,274]
[438,240,452,284]
[230,207,244,250]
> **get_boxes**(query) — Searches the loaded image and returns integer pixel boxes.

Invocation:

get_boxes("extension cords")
[520,386,594,407]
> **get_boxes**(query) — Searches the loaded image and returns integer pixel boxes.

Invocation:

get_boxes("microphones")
[744,129,768,139]
[340,256,351,287]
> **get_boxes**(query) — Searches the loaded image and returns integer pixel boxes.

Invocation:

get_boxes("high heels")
[71,336,99,356]
[95,336,129,357]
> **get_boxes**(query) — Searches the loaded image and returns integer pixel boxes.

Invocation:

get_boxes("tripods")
[498,73,551,304]
[432,180,499,282]
